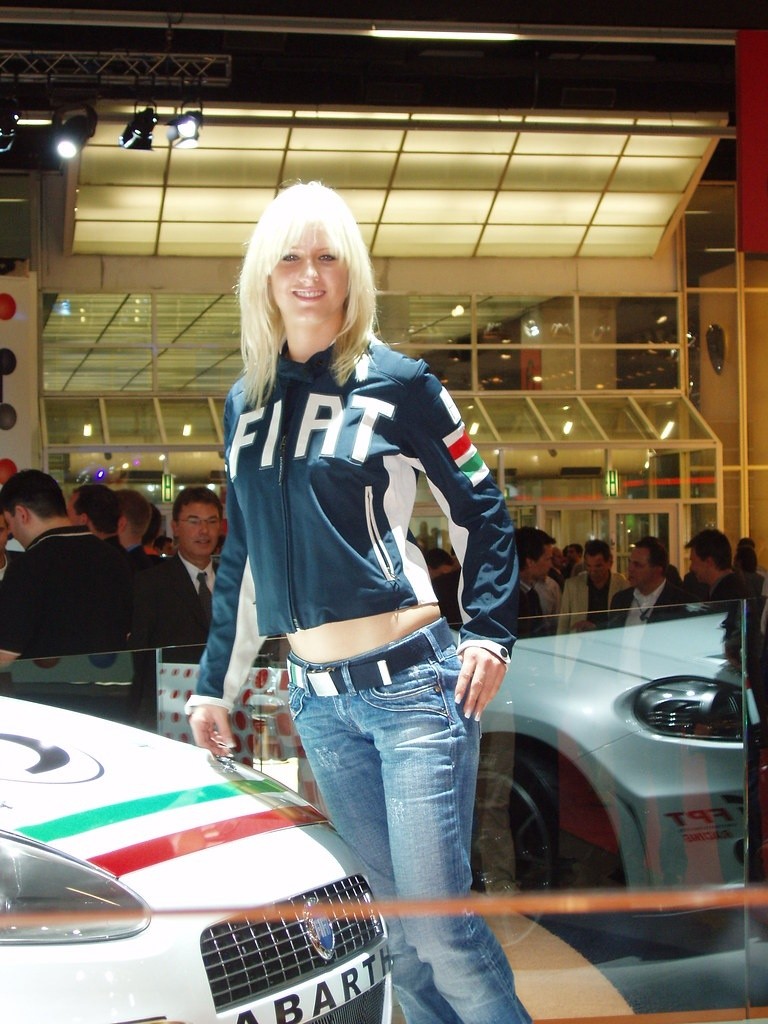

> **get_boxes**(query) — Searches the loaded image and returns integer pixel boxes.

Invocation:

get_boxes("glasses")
[179,517,221,526]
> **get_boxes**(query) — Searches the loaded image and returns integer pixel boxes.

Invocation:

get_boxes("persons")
[186,181,532,1023]
[0,471,768,758]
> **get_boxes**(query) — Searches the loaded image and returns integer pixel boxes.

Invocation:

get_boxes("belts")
[287,617,454,696]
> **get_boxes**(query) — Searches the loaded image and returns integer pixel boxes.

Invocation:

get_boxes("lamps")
[165,71,205,150]
[0,72,24,155]
[45,70,103,167]
[123,73,159,151]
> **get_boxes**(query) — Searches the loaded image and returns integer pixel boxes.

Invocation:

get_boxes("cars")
[442,605,746,959]
[0,683,399,1024]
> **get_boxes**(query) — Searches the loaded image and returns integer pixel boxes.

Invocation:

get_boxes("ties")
[196,573,212,626]
